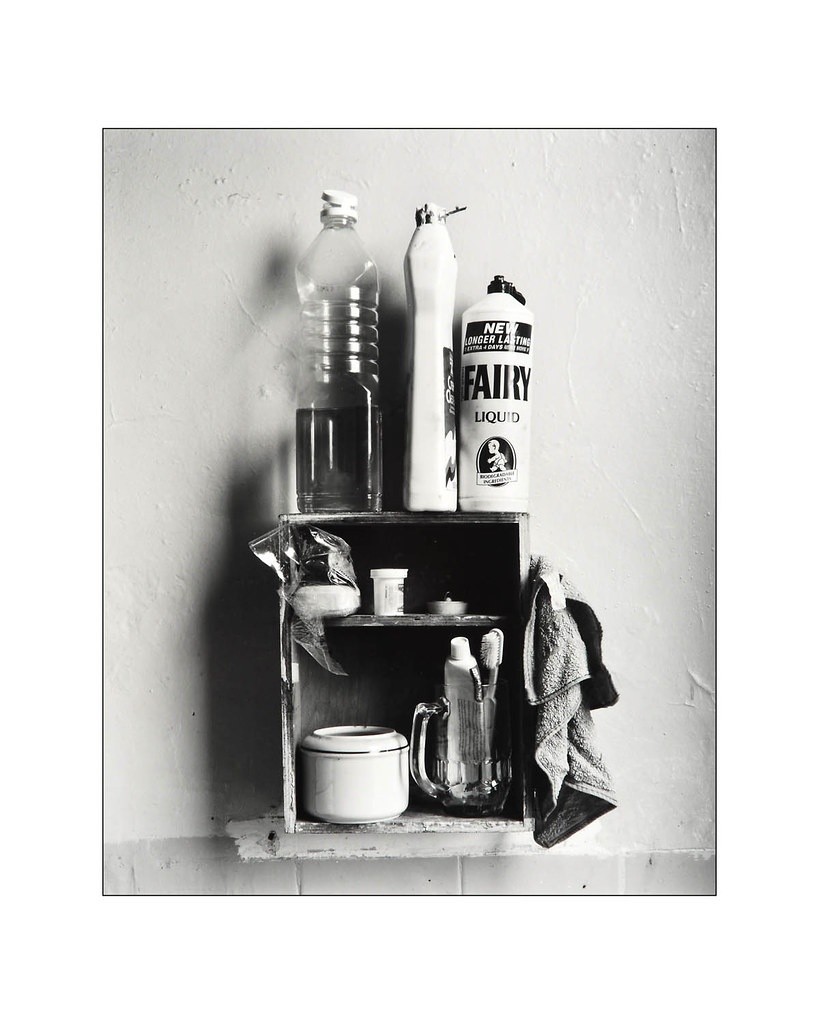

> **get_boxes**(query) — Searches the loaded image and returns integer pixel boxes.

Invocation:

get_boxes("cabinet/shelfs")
[279,511,537,836]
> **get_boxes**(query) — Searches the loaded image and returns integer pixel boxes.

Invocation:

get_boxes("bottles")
[458,275,535,513]
[295,190,383,513]
[402,202,466,513]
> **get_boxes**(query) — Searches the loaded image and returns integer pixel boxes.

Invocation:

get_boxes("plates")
[302,725,408,753]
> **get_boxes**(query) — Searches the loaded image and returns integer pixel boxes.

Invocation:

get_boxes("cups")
[299,748,409,823]
[370,569,408,616]
[409,678,511,815]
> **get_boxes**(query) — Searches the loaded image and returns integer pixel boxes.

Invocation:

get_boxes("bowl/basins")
[428,601,468,616]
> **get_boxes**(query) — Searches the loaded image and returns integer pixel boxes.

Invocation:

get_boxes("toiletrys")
[442,637,492,800]
[402,200,469,514]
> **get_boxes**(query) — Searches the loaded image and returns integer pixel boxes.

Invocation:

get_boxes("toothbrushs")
[478,627,503,746]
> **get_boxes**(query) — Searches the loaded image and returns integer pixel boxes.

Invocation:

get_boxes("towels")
[522,553,621,852]
